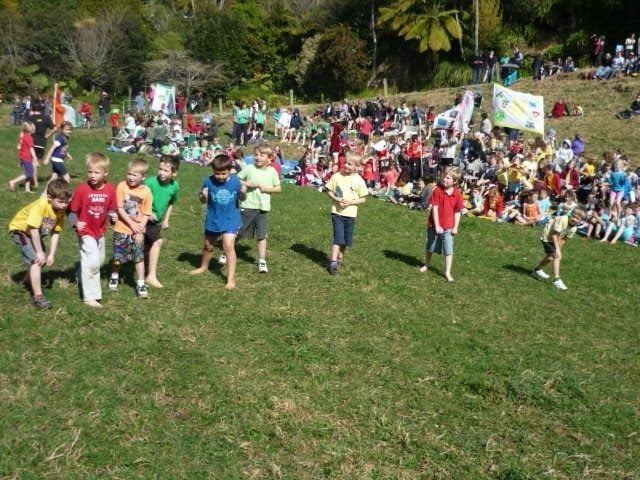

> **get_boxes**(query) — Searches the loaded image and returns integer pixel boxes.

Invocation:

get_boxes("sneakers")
[257,259,270,274]
[337,252,344,263]
[532,269,550,280]
[218,253,227,265]
[32,294,52,310]
[328,261,338,276]
[135,283,149,300]
[552,278,568,291]
[107,276,120,293]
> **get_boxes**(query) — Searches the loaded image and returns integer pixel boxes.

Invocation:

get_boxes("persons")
[468,32,639,86]
[10,85,640,309]
